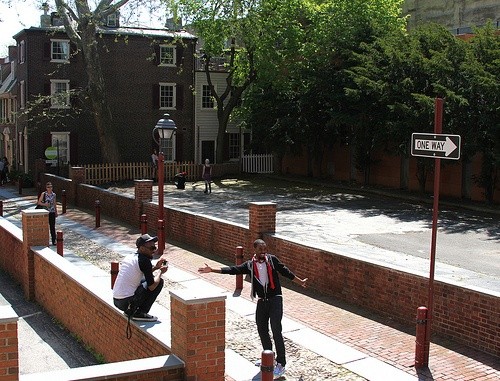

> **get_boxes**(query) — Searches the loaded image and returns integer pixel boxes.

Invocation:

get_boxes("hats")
[133,233,158,244]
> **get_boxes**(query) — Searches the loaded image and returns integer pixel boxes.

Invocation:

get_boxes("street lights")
[151,112,178,255]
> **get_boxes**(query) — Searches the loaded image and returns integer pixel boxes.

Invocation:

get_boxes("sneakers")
[273,363,286,380]
[124,309,159,322]
[254,358,262,367]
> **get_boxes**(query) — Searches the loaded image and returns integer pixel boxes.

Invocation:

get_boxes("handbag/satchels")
[33,203,43,210]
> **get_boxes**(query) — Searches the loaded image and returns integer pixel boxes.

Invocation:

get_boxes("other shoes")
[52,242,59,247]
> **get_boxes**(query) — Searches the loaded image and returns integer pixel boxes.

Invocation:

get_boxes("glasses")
[46,185,52,189]
[143,244,157,251]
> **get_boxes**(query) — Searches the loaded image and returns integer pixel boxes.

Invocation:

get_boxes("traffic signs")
[411,132,461,160]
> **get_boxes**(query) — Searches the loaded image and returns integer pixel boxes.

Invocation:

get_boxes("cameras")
[163,261,167,266]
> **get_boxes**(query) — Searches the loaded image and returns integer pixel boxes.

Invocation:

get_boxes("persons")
[0,158,5,185]
[37,181,57,245]
[174,172,187,189]
[198,239,309,376]
[112,233,168,321]
[202,159,213,195]
[3,156,9,184]
[151,149,158,182]
